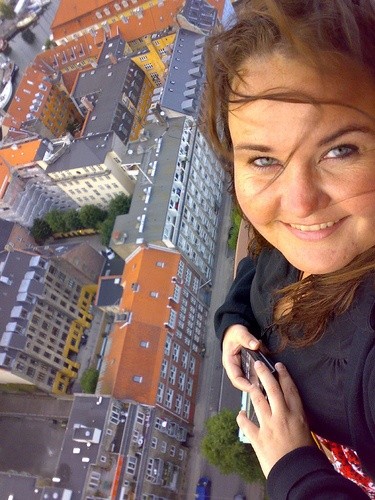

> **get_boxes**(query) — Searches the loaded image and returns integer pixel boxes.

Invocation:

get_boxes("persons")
[198,0,374,499]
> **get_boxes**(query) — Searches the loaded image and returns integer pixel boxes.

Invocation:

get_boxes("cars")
[194,475,211,500]
